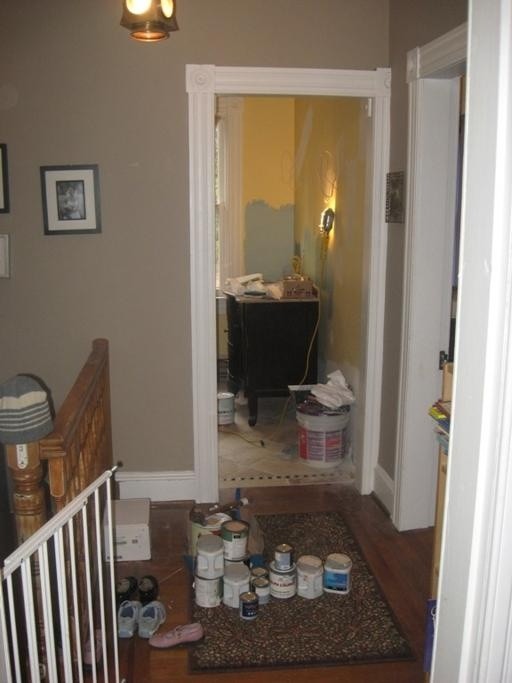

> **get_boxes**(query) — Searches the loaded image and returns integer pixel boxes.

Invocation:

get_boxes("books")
[427,399,452,458]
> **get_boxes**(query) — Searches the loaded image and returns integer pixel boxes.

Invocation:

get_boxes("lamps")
[120,0,179,43]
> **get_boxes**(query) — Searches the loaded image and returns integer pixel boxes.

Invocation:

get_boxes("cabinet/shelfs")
[223,279,320,428]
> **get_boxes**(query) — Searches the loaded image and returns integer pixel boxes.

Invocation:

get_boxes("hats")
[0,376,54,444]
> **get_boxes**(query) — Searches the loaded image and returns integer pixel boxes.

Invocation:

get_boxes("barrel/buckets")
[297,404,351,468]
[197,534,224,579]
[223,562,250,608]
[298,555,323,598]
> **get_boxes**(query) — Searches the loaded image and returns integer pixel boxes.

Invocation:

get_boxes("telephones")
[321,208,334,231]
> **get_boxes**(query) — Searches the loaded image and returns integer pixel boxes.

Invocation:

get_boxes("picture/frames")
[39,164,102,236]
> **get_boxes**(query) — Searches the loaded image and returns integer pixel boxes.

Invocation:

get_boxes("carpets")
[186,510,418,674]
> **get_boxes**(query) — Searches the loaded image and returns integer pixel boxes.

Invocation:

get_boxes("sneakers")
[149,623,204,648]
[117,577,137,604]
[138,575,159,606]
[83,629,102,665]
[118,600,142,638]
[138,601,167,638]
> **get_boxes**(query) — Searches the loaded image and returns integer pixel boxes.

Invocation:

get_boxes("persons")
[57,180,85,219]
